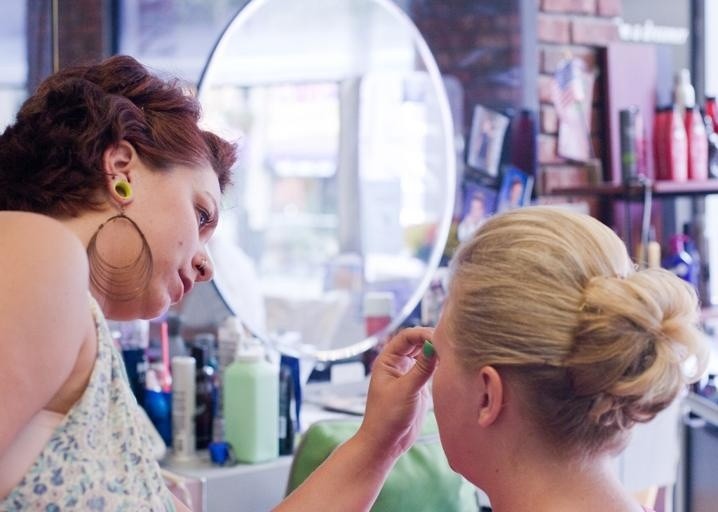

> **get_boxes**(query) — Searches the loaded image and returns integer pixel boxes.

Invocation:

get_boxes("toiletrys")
[141,368,170,445]
[651,66,718,182]
[190,347,215,450]
[278,363,298,455]
[617,106,640,182]
[171,356,197,460]
[637,218,701,294]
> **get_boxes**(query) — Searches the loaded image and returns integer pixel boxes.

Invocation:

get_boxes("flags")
[550,57,586,106]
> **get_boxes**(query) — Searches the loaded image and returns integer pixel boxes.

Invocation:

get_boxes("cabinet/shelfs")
[551,176,717,343]
[687,377,717,512]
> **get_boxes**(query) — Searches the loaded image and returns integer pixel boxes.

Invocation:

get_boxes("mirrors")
[194,0,458,362]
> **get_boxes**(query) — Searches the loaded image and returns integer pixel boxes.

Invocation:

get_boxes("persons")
[457,195,489,244]
[0,55,436,512]
[499,175,524,211]
[429,204,708,512]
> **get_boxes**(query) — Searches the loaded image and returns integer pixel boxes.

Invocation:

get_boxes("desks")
[165,388,686,512]
